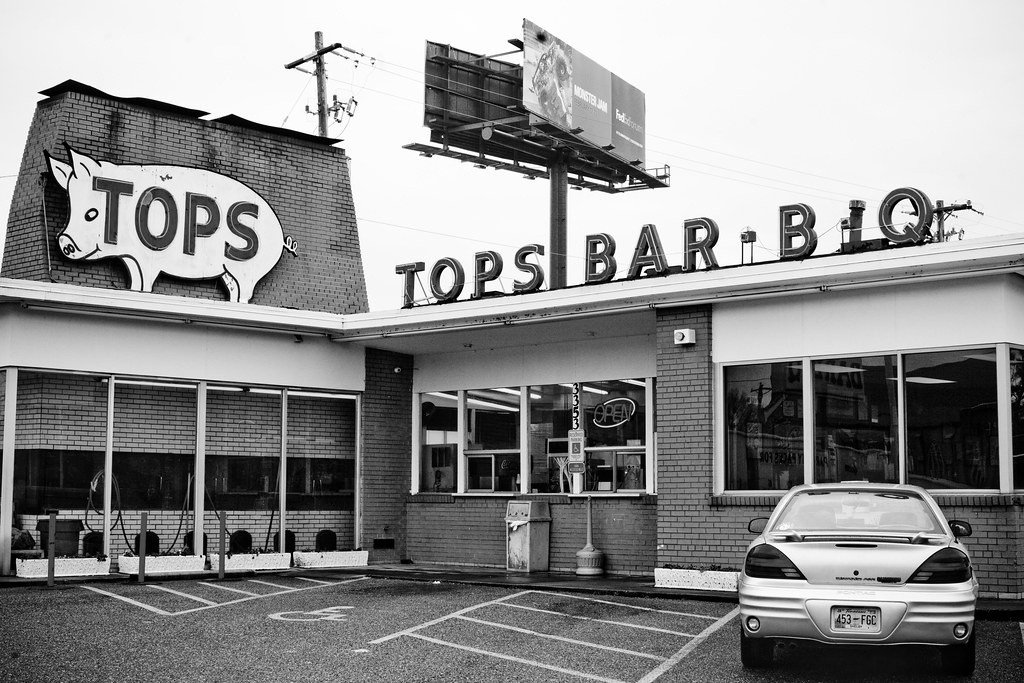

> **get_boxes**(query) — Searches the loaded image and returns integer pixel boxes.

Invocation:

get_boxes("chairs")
[879,512,917,525]
[793,504,836,527]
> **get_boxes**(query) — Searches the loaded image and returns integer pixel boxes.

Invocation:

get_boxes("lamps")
[559,382,610,395]
[425,388,542,412]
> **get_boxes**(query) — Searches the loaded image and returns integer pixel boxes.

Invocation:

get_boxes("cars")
[737,482,978,677]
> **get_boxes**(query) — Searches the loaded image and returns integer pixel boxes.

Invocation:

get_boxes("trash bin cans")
[506,500,552,573]
[36,519,82,557]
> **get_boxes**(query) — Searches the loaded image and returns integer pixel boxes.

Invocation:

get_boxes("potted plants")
[118,551,206,574]
[210,546,291,570]
[15,552,111,579]
[293,547,369,568]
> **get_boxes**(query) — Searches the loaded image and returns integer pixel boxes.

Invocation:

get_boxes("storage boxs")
[598,482,613,491]
